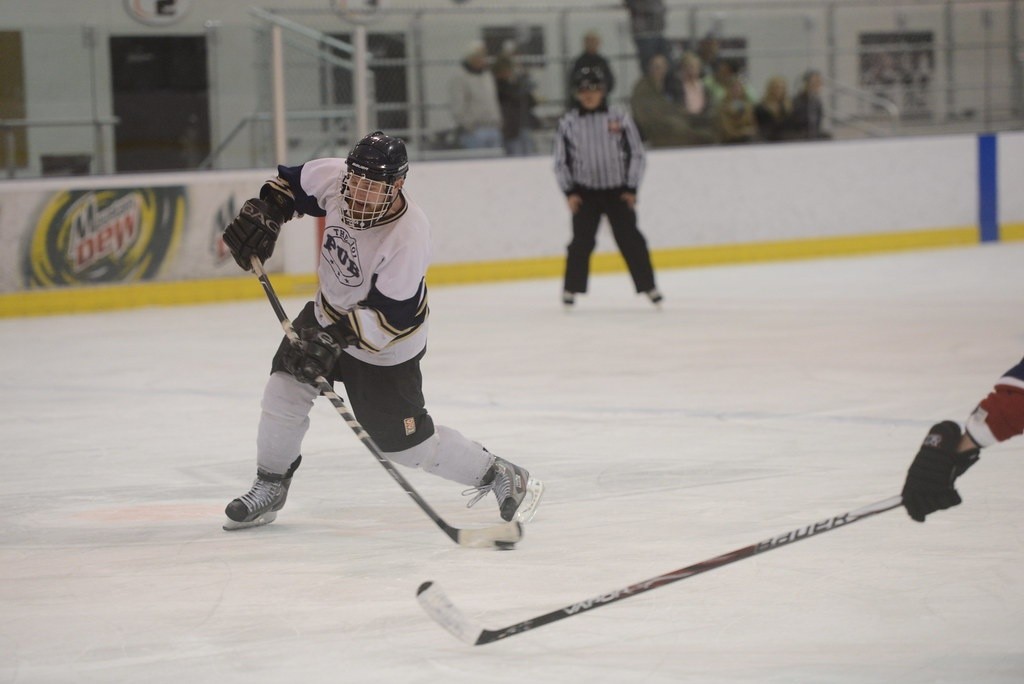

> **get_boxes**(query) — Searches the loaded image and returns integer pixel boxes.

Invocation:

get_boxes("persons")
[559,63,663,307]
[631,38,758,146]
[573,32,614,94]
[222,131,546,531]
[757,75,794,141]
[792,71,831,140]
[443,42,536,155]
[902,354,1024,523]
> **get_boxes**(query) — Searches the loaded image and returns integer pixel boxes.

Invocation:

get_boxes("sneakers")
[462,447,546,522]
[223,455,302,531]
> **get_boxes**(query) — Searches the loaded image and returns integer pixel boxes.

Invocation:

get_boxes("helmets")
[572,67,607,91]
[347,131,409,178]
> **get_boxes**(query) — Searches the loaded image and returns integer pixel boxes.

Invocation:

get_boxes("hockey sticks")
[249,250,522,548]
[414,490,903,647]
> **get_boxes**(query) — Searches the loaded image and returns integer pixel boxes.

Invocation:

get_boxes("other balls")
[493,539,517,550]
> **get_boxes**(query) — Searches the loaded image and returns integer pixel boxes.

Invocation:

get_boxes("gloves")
[902,420,980,523]
[222,198,292,271]
[295,323,359,384]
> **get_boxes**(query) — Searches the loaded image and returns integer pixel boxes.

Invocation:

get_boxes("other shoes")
[648,289,661,302]
[563,292,573,304]
